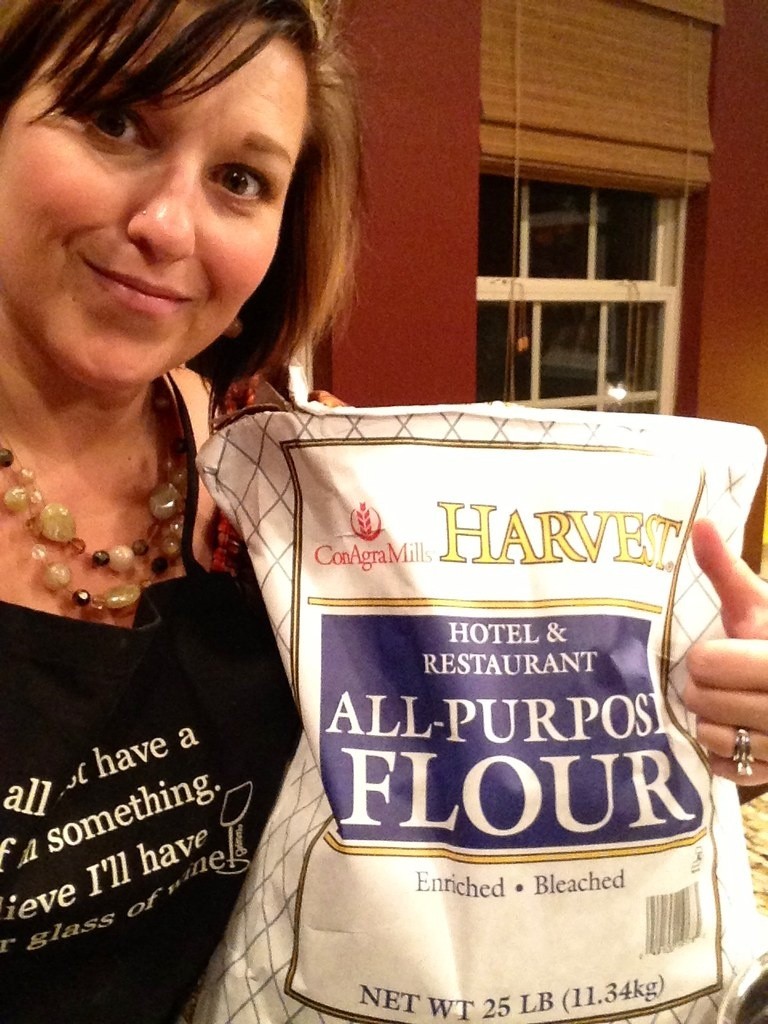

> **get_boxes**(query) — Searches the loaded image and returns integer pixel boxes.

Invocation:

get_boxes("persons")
[1,0,768,1023]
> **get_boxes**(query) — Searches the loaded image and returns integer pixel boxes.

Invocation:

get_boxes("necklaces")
[0,379,191,611]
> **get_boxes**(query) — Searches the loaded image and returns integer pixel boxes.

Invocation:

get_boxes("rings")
[732,724,753,777]
